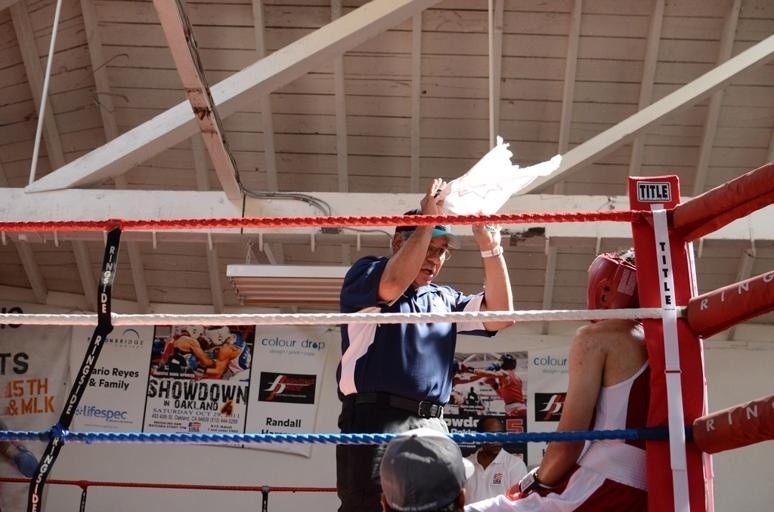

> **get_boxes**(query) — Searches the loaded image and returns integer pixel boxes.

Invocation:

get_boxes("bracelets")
[479,245,504,259]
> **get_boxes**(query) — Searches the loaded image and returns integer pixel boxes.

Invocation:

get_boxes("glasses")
[424,246,451,261]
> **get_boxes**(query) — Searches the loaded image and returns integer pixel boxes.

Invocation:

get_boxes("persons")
[464,386,478,406]
[464,246,651,512]
[456,353,527,415]
[449,358,507,405]
[153,324,252,381]
[333,178,516,512]
[461,415,526,508]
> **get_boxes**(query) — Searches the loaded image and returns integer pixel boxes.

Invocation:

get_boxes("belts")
[355,391,444,418]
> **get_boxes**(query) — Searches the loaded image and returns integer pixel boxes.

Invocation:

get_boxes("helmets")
[181,326,204,339]
[586,252,640,323]
[204,326,231,346]
[498,354,517,370]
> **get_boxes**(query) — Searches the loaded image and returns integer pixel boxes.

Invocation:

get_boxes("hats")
[400,228,462,250]
